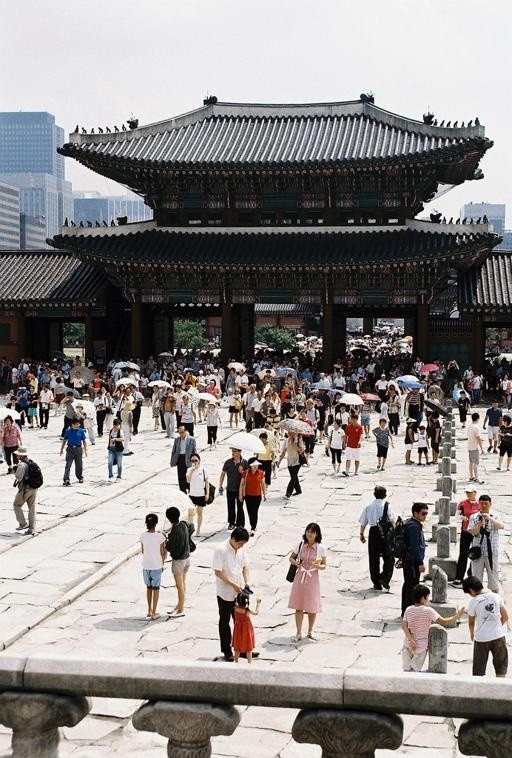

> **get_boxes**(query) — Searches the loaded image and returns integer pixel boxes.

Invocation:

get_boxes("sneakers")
[108,478,120,484]
[228,524,256,536]
[154,423,174,438]
[16,523,35,535]
[453,579,462,585]
[468,476,484,484]
[28,425,47,429]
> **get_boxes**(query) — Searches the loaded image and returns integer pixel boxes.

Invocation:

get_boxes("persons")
[163,507,195,618]
[359,485,396,588]
[463,575,510,676]
[455,484,482,583]
[466,494,505,593]
[230,586,262,662]
[286,521,327,643]
[403,584,465,671]
[139,514,165,620]
[388,502,430,617]
[213,527,261,662]
[1,323,512,534]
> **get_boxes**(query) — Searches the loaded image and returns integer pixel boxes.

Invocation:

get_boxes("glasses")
[191,459,198,462]
[422,512,428,515]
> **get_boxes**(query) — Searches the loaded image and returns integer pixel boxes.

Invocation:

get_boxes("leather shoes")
[63,479,83,485]
[224,652,259,662]
[374,574,390,590]
[146,608,185,620]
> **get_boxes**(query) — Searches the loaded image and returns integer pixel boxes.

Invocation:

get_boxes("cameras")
[478,514,482,522]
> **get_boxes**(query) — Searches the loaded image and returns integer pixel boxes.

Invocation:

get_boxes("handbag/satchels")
[286,555,302,582]
[299,451,307,465]
[466,546,481,561]
[204,483,215,504]
[189,538,196,552]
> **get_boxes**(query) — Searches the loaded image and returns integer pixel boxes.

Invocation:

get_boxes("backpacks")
[22,459,42,489]
[376,502,412,568]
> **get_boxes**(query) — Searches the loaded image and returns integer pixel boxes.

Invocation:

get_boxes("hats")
[82,394,90,397]
[406,418,417,423]
[13,446,28,456]
[464,484,477,493]
[249,460,262,466]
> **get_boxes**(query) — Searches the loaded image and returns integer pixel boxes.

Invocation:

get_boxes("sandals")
[293,633,320,643]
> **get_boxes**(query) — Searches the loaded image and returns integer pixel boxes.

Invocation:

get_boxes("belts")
[68,446,81,447]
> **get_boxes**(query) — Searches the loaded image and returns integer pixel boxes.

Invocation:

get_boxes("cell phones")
[298,559,303,565]
